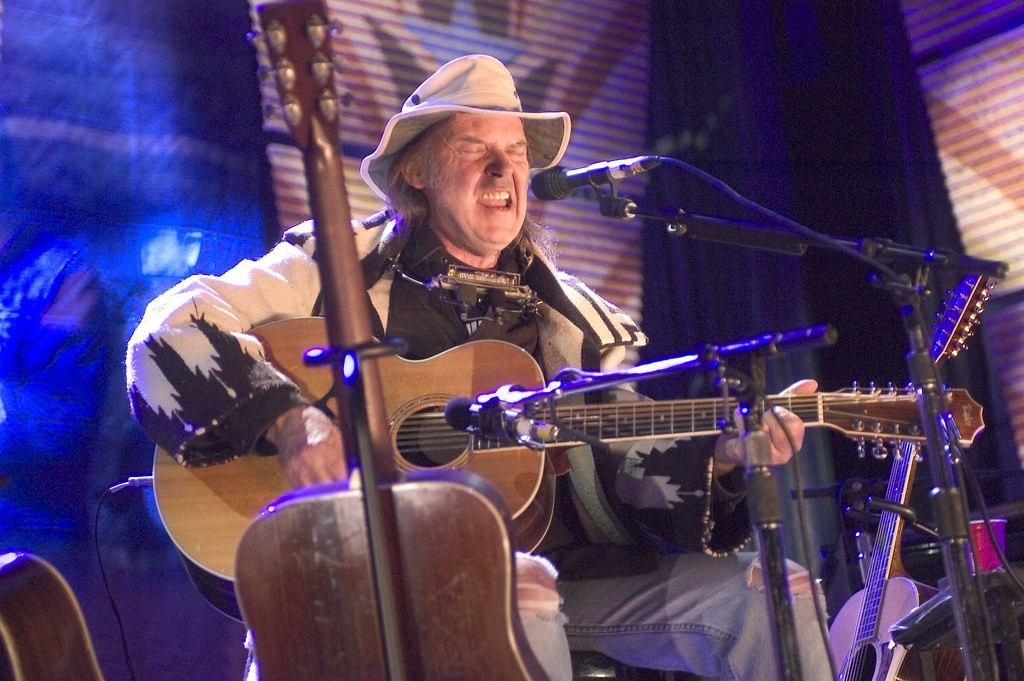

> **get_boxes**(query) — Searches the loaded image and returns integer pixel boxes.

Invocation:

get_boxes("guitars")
[226,1,548,681]
[820,268,998,681]
[1,550,104,680]
[148,313,989,588]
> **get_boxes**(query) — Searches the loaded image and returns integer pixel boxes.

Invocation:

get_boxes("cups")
[969,520,1005,575]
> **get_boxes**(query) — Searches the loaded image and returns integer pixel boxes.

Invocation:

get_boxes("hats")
[360,53,572,206]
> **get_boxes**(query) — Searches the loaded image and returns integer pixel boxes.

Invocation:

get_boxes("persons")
[124,51,821,681]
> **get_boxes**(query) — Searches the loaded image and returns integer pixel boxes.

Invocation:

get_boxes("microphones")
[444,398,576,443]
[529,155,662,201]
